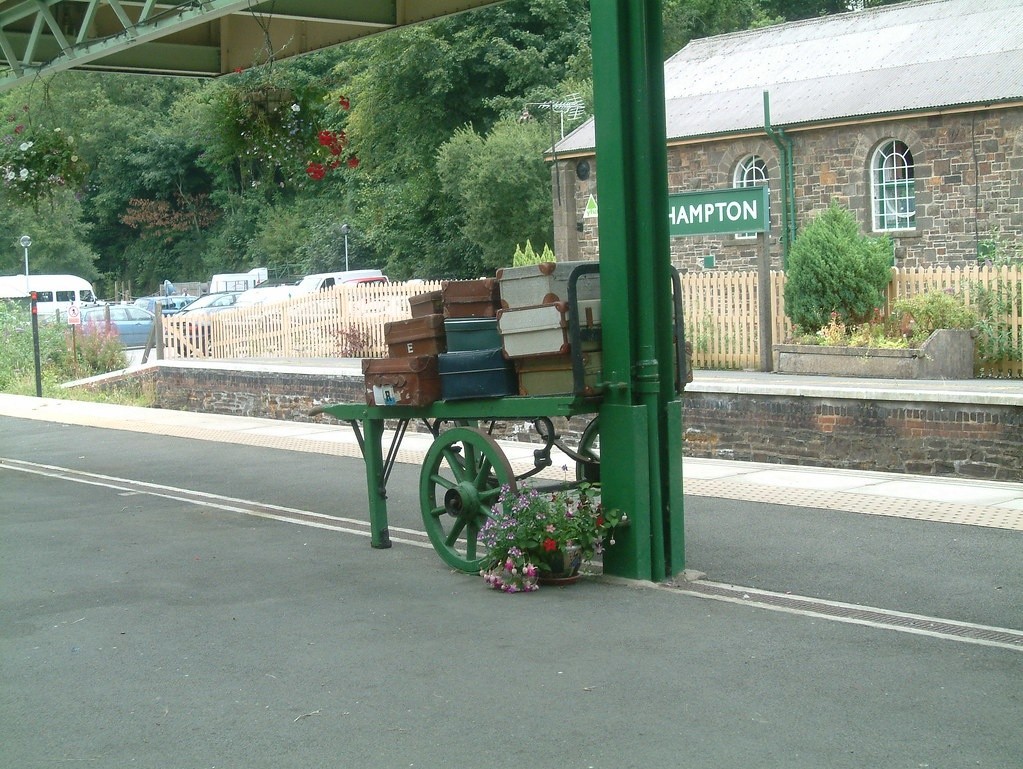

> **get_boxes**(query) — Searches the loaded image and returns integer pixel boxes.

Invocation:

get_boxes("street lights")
[518,102,564,140]
[19,235,32,277]
[340,224,351,271]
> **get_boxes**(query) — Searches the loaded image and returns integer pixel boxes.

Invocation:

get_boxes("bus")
[13,274,97,322]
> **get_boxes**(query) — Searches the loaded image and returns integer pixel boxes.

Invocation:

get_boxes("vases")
[525,543,582,586]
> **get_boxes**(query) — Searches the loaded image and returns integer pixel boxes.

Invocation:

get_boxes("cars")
[45,302,157,348]
[170,290,244,357]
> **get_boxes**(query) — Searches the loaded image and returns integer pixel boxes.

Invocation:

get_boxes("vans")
[287,270,381,300]
[135,295,200,318]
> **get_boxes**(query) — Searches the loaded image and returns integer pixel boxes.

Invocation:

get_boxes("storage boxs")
[443,317,505,352]
[437,349,517,401]
[383,314,447,359]
[409,288,444,321]
[361,355,443,408]
[440,277,500,319]
[494,298,601,361]
[510,351,602,398]
[495,259,601,310]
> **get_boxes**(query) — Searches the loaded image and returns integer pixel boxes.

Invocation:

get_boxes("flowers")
[475,464,632,594]
